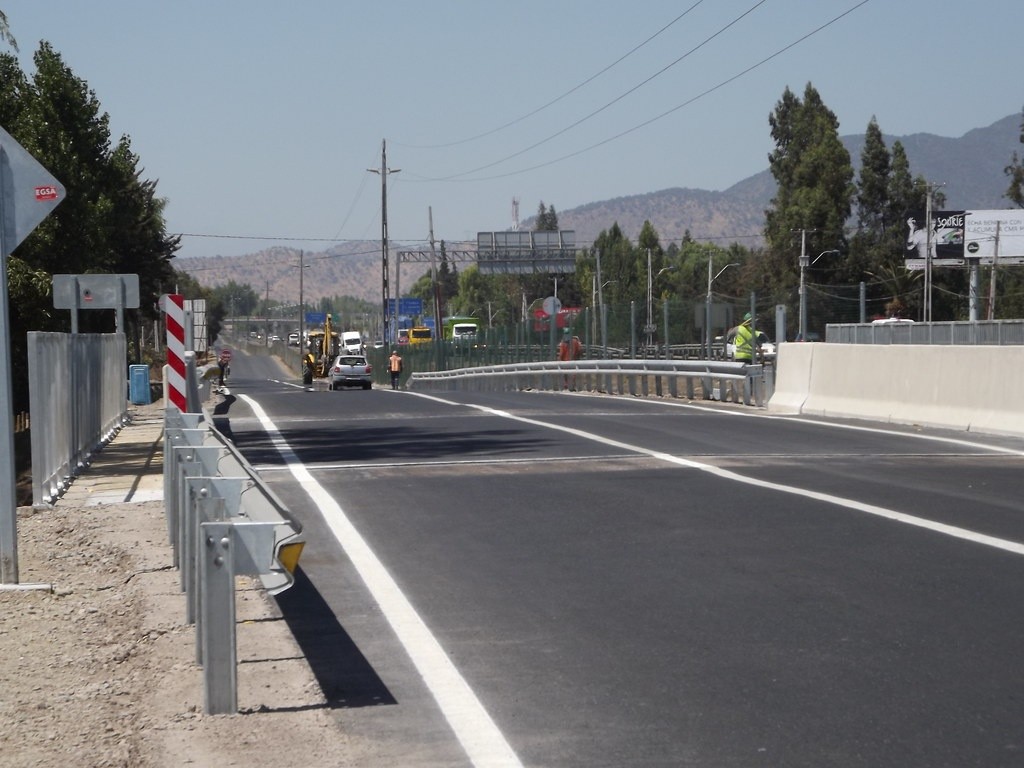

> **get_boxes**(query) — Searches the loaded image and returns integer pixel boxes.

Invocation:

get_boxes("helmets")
[392,351,398,355]
[743,313,753,321]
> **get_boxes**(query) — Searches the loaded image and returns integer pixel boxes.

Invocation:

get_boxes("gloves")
[387,370,389,373]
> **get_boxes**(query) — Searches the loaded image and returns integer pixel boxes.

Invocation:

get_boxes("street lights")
[644,265,677,346]
[593,273,620,344]
[488,301,506,329]
[798,248,846,342]
[707,262,741,360]
[521,289,545,323]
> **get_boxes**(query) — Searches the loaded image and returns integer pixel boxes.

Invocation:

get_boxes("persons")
[387,350,401,390]
[220,349,231,367]
[559,328,581,388]
[727,313,762,396]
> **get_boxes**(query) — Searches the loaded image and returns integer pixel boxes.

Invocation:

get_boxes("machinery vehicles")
[301,313,340,384]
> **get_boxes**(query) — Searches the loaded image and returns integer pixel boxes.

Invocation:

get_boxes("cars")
[373,340,384,349]
[713,332,777,362]
[327,354,372,390]
[795,332,824,343]
[250,331,300,348]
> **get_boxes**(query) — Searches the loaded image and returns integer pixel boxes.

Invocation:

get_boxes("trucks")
[398,315,480,346]
[339,330,364,356]
